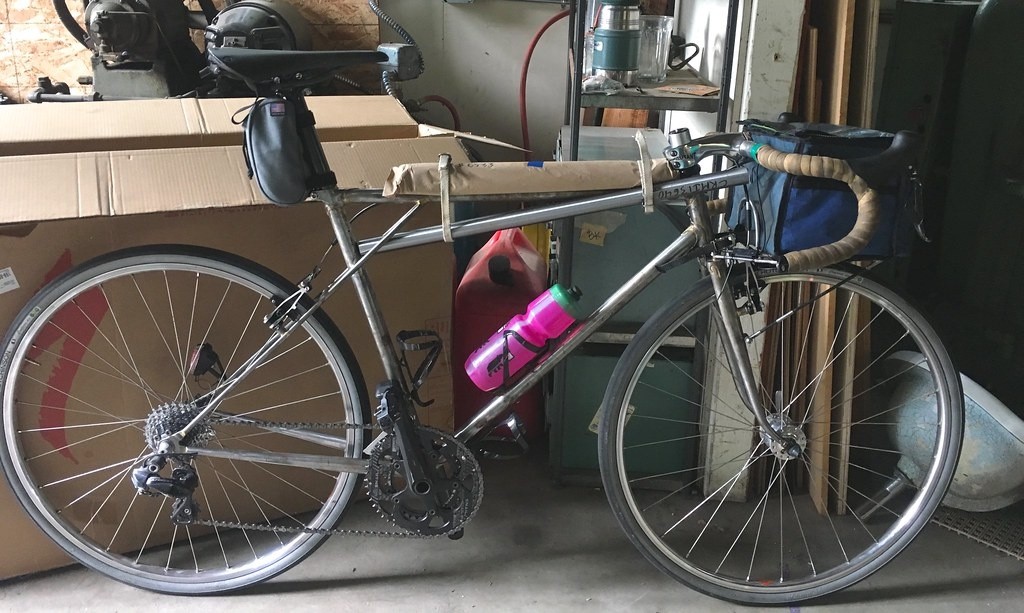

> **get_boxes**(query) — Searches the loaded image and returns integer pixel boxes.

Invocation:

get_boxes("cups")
[638,16,675,87]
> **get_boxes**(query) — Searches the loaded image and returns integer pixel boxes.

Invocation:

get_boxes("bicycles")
[0,47,965,607]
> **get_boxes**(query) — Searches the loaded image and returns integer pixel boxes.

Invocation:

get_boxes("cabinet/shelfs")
[544,0,738,494]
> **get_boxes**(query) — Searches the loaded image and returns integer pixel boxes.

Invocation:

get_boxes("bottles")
[595,0,642,87]
[464,284,584,392]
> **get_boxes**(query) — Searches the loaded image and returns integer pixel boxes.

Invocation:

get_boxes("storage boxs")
[0,93,482,586]
